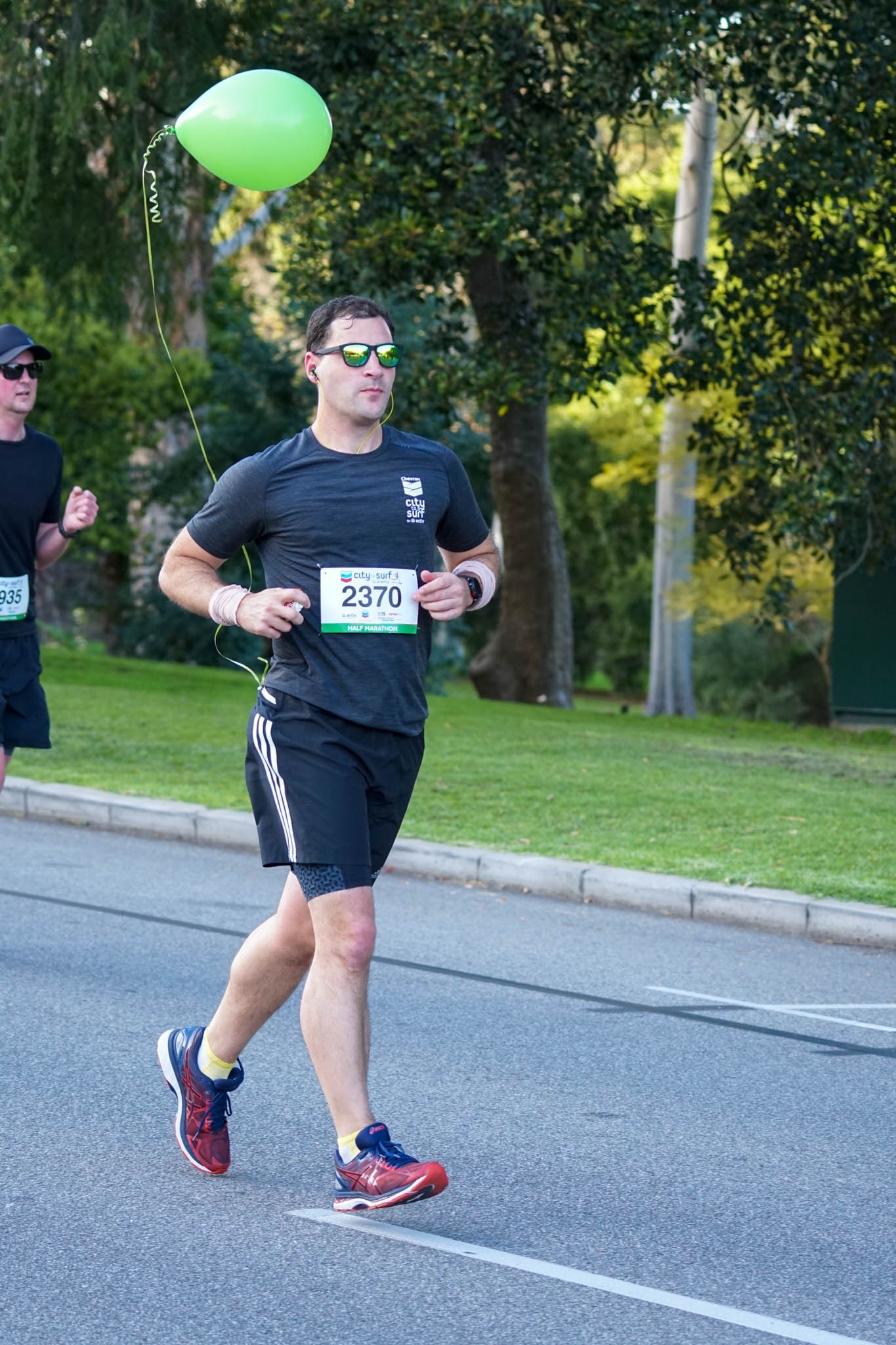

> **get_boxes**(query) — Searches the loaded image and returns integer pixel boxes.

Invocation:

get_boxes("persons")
[156,296,507,1217]
[0,325,100,791]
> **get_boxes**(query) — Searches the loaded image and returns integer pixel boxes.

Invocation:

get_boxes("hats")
[0,323,52,363]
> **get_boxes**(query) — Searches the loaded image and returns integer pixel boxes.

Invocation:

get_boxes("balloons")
[176,69,333,193]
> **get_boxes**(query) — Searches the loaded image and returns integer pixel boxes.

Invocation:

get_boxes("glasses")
[311,342,404,367]
[0,362,44,380]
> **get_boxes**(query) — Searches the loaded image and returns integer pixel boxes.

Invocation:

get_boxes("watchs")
[458,574,482,609]
[56,517,81,539]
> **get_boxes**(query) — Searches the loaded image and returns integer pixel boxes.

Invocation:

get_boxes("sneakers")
[157,1025,245,1176]
[332,1120,447,1212]
